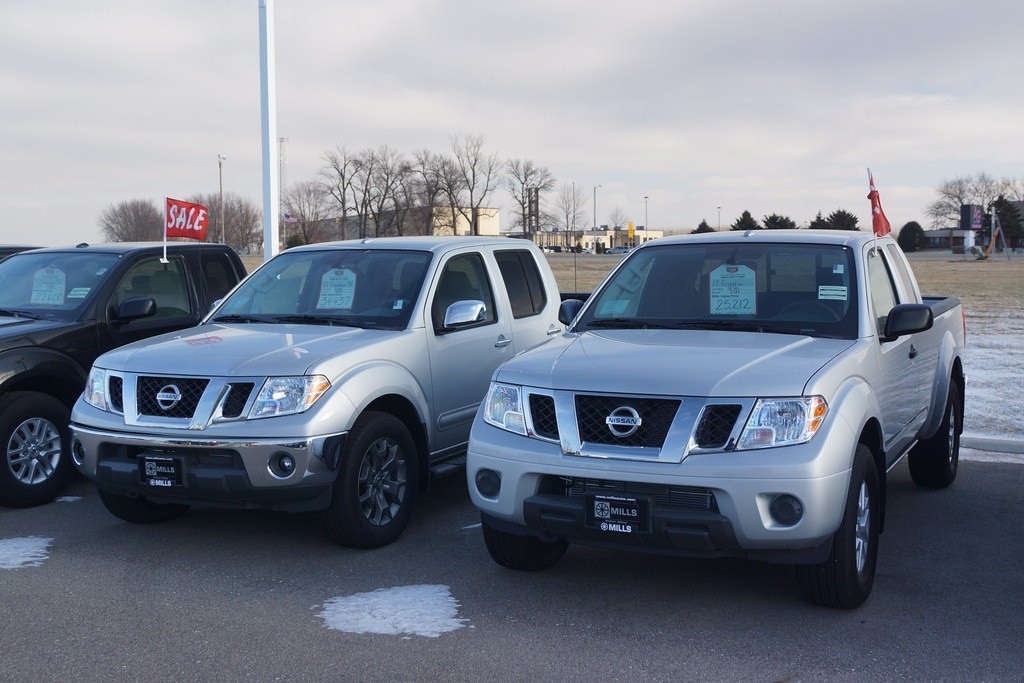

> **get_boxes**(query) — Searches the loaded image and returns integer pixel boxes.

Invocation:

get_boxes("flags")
[284,214,298,223]
[166,198,208,241]
[867,168,890,237]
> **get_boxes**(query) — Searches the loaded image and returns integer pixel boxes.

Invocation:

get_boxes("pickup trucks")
[465,227,968,614]
[63,236,580,553]
[2,241,255,510]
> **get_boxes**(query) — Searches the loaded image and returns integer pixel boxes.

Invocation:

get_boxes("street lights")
[276,134,290,258]
[643,196,650,243]
[217,151,227,250]
[593,184,601,255]
[717,206,722,233]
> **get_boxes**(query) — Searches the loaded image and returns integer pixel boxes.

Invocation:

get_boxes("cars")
[577,247,596,255]
[549,245,565,251]
[538,245,554,254]
[561,246,578,253]
[605,245,633,254]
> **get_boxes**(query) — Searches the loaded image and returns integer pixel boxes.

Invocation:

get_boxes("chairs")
[401,261,476,319]
[145,270,188,310]
